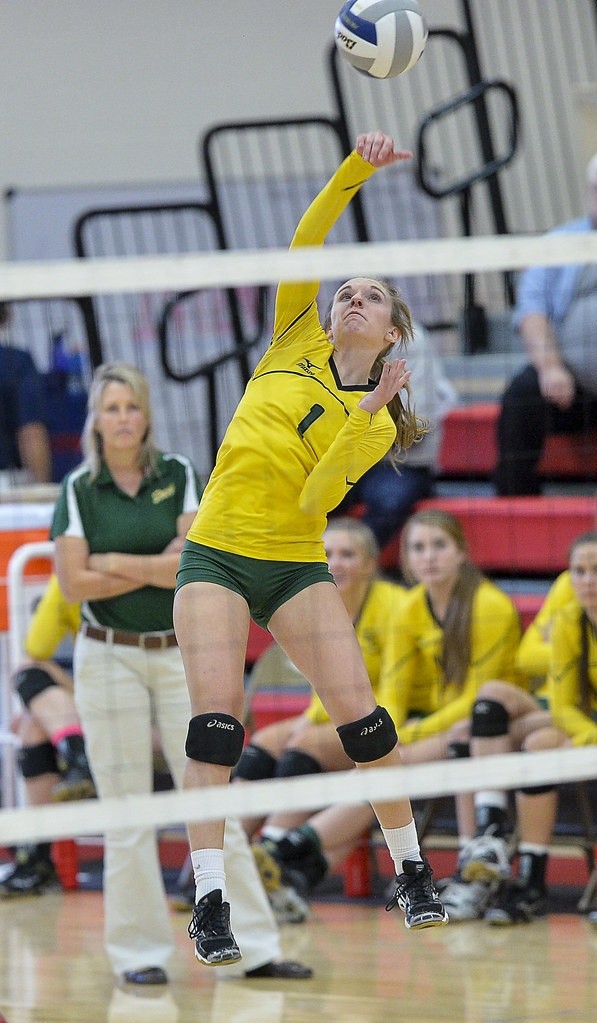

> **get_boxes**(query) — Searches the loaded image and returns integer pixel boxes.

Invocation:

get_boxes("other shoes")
[439,880,489,921]
[50,767,97,802]
[0,858,63,900]
[245,961,313,979]
[456,840,500,884]
[252,841,311,924]
[124,967,168,985]
[487,881,548,924]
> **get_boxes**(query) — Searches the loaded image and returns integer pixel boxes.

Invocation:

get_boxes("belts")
[79,625,178,649]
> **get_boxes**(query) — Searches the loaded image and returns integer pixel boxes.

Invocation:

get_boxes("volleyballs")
[334,0,429,80]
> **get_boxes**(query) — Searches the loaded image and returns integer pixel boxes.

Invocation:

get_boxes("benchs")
[50,309,597,894]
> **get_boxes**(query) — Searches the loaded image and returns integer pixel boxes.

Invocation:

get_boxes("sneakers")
[188,889,242,966]
[384,851,448,931]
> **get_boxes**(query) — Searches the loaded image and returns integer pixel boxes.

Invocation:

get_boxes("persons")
[0,506,597,925]
[173,133,449,964]
[47,363,319,983]
[0,303,54,488]
[495,153,597,497]
